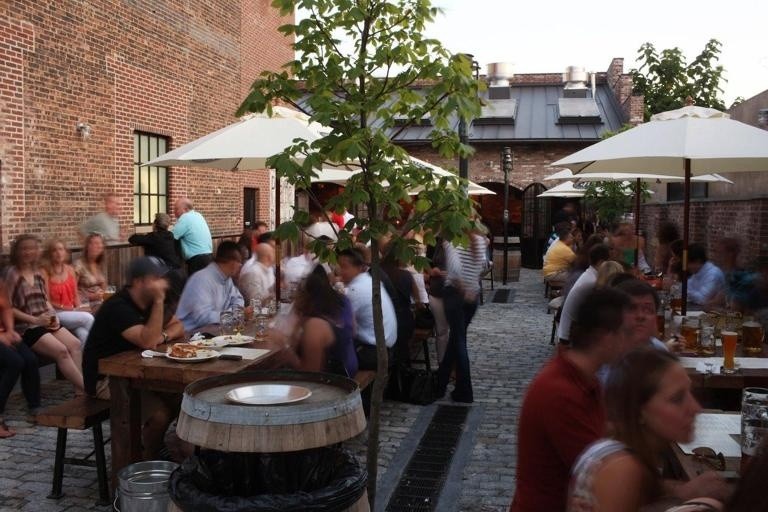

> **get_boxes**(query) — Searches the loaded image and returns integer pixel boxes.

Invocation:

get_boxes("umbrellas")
[536,180,656,198]
[138,106,376,307]
[543,168,735,267]
[284,142,497,197]
[550,105,768,316]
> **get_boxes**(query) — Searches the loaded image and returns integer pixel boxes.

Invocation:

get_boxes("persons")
[507,219,767,511]
[1,195,494,439]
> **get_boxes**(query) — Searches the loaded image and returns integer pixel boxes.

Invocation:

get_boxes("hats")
[125,255,174,279]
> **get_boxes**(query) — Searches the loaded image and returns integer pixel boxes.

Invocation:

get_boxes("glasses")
[693,448,725,474]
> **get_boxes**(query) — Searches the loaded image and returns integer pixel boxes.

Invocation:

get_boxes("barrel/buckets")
[114,461,181,512]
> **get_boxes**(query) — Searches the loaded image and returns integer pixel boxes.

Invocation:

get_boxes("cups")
[48,311,58,329]
[680,324,700,354]
[255,316,268,341]
[219,311,234,336]
[233,306,245,331]
[740,386,768,440]
[741,418,767,475]
[697,328,716,355]
[671,295,682,311]
[742,320,766,352]
[720,326,737,374]
[104,286,116,299]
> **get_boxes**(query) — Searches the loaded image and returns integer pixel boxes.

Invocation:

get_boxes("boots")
[451,377,473,403]
[438,369,450,399]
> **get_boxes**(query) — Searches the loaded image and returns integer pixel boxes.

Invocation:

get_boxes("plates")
[211,335,254,345]
[188,339,229,349]
[224,385,312,406]
[165,349,220,362]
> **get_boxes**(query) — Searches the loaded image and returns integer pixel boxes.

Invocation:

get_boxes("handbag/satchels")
[382,367,450,406]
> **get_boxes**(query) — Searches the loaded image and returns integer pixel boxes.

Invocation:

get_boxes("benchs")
[478,267,493,305]
[355,365,375,389]
[25,394,112,506]
[548,296,563,351]
[407,327,432,371]
[543,277,566,314]
